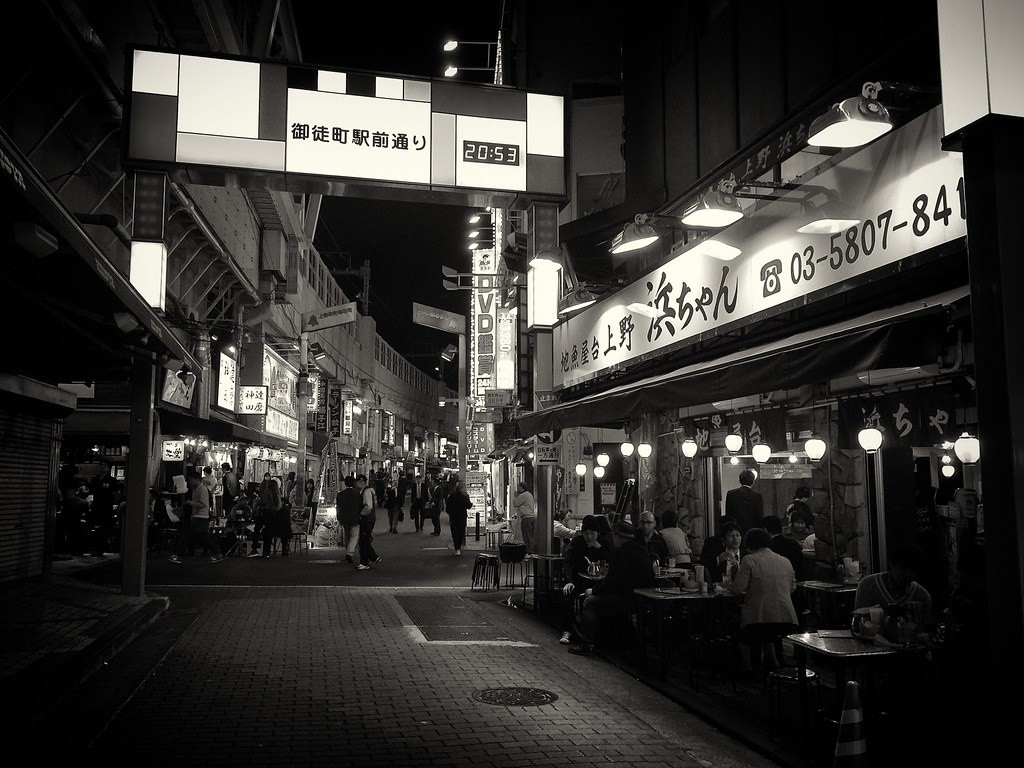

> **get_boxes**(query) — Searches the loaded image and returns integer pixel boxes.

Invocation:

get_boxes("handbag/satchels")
[398,508,404,521]
[410,505,415,519]
[423,505,436,518]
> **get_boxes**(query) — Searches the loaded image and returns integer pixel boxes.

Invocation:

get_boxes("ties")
[730,554,739,587]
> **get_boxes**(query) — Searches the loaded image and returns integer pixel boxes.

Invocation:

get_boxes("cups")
[695,566,704,583]
[702,582,707,593]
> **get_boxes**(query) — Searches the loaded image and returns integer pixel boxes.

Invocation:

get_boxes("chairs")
[157,504,193,555]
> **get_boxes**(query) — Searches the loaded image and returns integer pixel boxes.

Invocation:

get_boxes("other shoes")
[246,550,259,558]
[455,549,460,557]
[168,555,182,564]
[430,532,440,536]
[346,555,353,564]
[569,643,592,655]
[393,530,398,534]
[208,552,223,563]
[559,631,572,645]
[389,528,392,533]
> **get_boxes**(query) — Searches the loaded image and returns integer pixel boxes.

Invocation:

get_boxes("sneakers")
[355,563,369,570]
[369,557,381,567]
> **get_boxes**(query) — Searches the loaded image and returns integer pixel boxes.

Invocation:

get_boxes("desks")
[787,629,906,725]
[578,571,683,607]
[794,580,858,631]
[634,588,747,684]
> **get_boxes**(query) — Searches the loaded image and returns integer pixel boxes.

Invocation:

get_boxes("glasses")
[723,533,741,540]
[640,520,654,525]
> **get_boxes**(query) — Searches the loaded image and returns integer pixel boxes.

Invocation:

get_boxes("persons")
[57,459,314,566]
[563,511,692,655]
[514,482,534,561]
[699,470,814,682]
[854,541,932,630]
[336,467,473,570]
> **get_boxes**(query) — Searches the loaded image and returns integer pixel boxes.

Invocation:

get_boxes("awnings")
[515,284,971,438]
[159,399,287,449]
[313,431,356,458]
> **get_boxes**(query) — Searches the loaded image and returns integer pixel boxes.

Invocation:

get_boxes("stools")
[611,610,819,757]
[471,553,535,607]
[210,526,226,554]
[288,532,308,554]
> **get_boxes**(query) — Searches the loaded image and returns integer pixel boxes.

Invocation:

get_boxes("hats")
[581,515,600,532]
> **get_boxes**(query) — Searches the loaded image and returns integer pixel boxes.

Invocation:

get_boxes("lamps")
[682,177,801,227]
[444,40,499,77]
[608,212,707,254]
[178,372,195,386]
[469,212,493,223]
[114,312,139,334]
[128,237,167,317]
[467,227,493,238]
[526,242,623,333]
[468,240,494,250]
[807,81,930,148]
[575,352,979,478]
[777,184,862,236]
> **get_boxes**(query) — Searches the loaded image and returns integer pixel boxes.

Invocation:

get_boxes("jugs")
[586,562,600,577]
